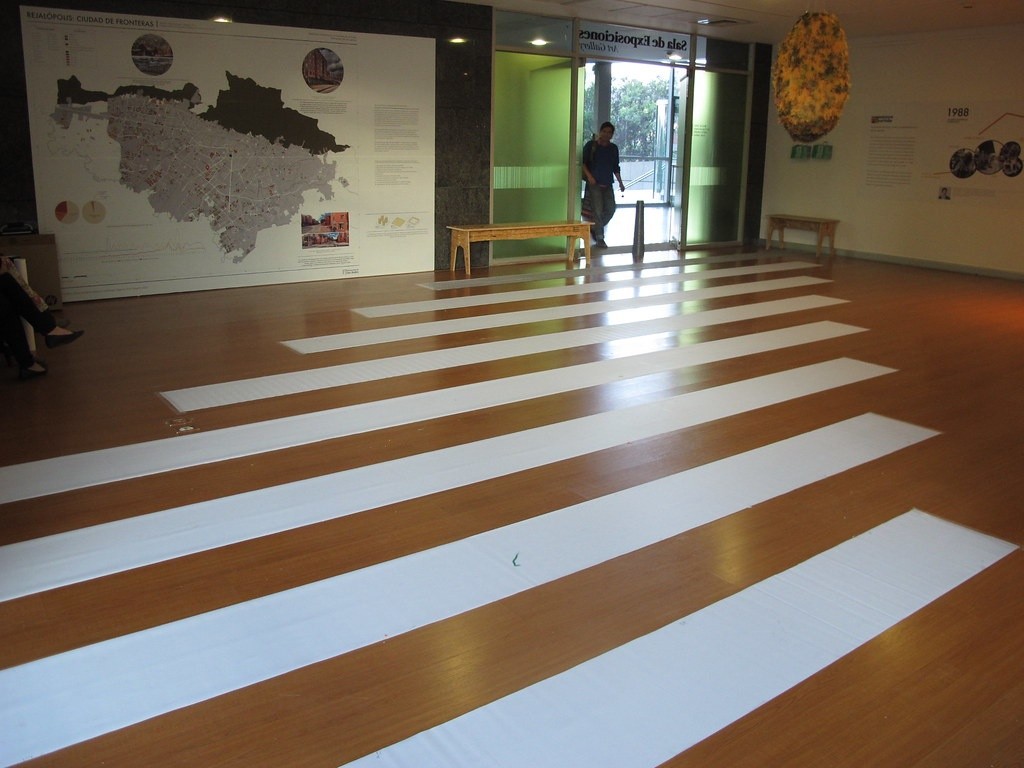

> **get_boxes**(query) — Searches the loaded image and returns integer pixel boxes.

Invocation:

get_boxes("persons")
[0,256,84,380]
[583,122,625,249]
[938,188,950,200]
[953,142,1022,178]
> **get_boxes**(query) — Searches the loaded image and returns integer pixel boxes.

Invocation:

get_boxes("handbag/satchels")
[582,141,597,182]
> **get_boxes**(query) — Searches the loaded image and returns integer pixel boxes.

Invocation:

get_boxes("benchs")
[444,219,596,275]
[764,214,840,257]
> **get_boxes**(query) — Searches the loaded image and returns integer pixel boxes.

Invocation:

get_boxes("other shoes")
[590,225,596,241]
[596,240,607,248]
[19,361,49,377]
[45,330,86,347]
